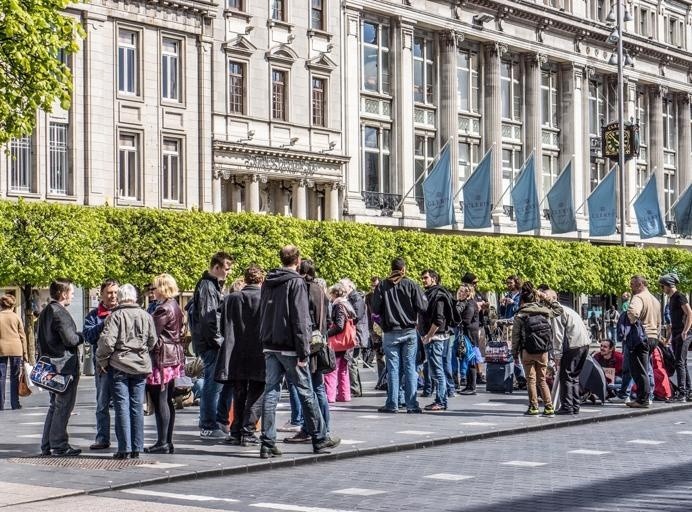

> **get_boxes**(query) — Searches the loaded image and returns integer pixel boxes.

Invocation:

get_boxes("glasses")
[660,285,666,288]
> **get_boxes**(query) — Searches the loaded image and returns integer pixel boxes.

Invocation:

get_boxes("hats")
[460,272,475,282]
[659,273,681,288]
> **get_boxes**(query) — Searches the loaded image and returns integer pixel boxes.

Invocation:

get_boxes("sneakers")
[524,391,692,415]
[40,442,174,460]
[201,425,342,459]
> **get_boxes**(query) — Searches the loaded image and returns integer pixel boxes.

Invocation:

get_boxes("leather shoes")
[378,392,448,413]
[460,389,476,395]
[458,387,467,392]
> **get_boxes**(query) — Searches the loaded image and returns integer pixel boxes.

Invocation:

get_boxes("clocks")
[600,116,641,163]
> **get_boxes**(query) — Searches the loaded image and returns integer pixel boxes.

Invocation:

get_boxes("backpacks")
[519,313,553,354]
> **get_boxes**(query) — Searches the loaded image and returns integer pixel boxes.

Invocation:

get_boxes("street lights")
[606,0,635,246]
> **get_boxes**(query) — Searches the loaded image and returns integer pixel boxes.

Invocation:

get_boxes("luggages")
[486,363,513,394]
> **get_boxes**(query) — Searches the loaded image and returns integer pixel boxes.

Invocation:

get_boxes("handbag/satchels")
[30,355,80,392]
[485,340,508,363]
[18,362,32,397]
[311,319,356,375]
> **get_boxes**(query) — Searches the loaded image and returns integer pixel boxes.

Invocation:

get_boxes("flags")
[421,144,456,229]
[673,182,692,236]
[587,167,617,236]
[463,151,491,228]
[633,170,667,239]
[510,152,541,232]
